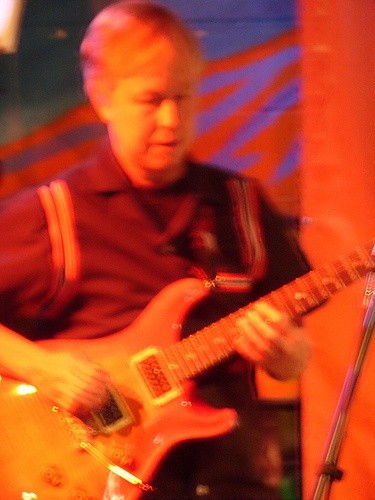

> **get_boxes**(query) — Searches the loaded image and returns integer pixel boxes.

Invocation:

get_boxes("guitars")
[0,240,375,500]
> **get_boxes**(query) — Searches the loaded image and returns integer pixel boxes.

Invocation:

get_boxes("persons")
[1,1,316,500]
